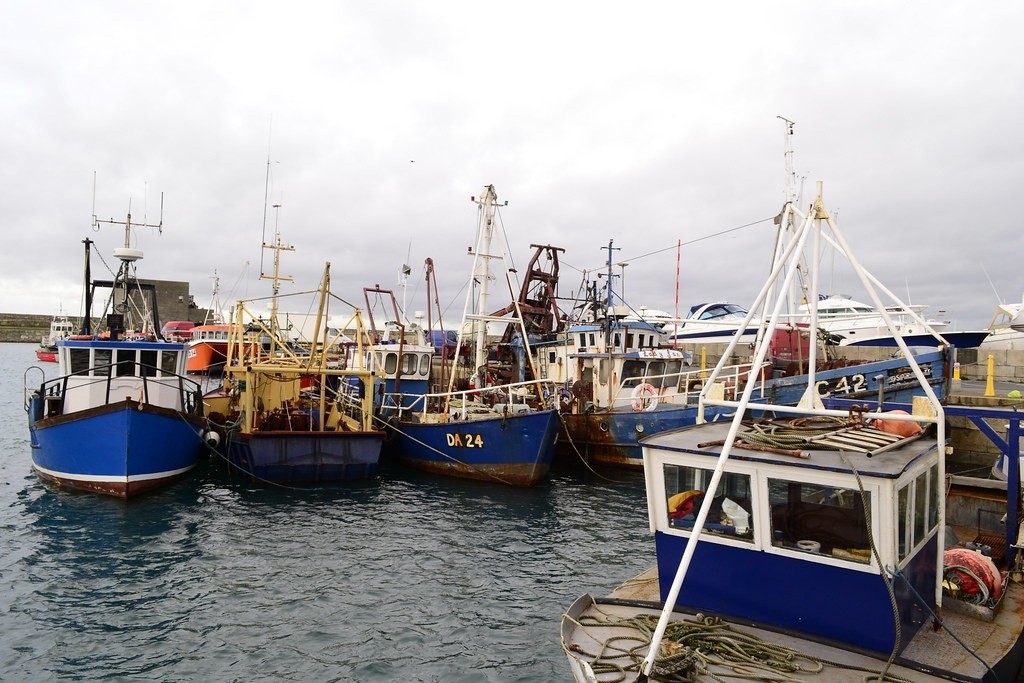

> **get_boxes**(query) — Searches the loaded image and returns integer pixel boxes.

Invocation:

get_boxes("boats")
[23,172,1024,683]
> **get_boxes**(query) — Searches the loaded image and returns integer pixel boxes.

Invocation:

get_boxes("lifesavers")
[631,381,659,412]
[470,371,492,397]
